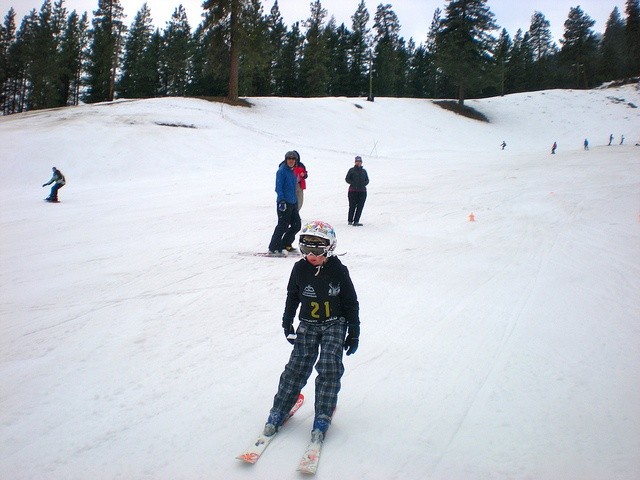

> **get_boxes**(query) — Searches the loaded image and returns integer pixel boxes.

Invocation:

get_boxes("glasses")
[300,240,328,256]
[356,161,362,162]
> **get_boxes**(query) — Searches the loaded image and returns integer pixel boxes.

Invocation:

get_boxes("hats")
[355,156,361,160]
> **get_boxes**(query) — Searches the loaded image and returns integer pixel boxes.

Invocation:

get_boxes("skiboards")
[235,395,324,474]
[239,251,348,257]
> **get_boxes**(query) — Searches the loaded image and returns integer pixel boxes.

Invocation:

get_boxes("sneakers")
[353,221,363,226]
[283,245,296,252]
[311,414,331,442]
[348,221,352,225]
[268,249,288,256]
[264,407,288,436]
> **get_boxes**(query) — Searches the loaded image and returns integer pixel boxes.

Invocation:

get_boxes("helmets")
[285,151,298,159]
[298,221,337,258]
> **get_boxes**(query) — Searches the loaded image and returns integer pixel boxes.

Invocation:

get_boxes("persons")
[551,142,558,153]
[43,167,65,201]
[265,152,300,257]
[500,140,506,148]
[608,134,614,145]
[620,134,624,144]
[265,222,359,443]
[584,139,589,150]
[279,150,308,211]
[346,157,368,226]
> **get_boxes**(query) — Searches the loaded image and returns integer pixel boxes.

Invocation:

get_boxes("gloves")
[282,319,295,344]
[345,326,360,356]
[278,201,287,212]
